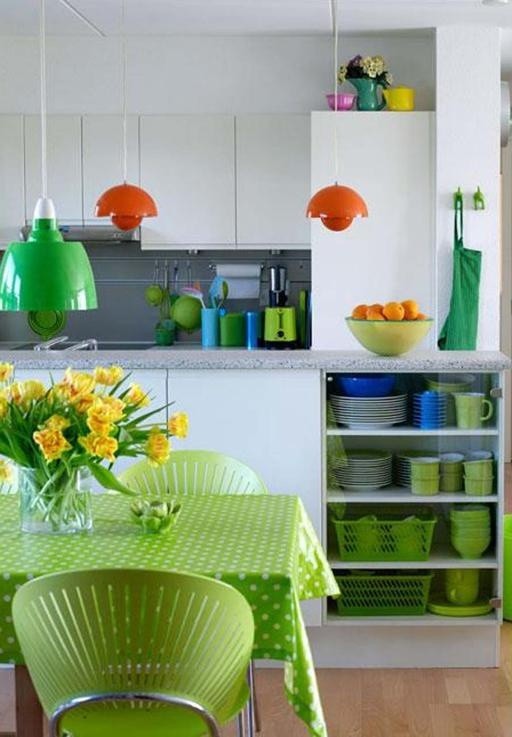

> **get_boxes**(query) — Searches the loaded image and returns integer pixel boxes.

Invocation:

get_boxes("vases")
[341,80,389,112]
[16,465,94,538]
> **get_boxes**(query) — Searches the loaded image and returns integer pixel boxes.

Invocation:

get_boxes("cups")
[388,514,422,542]
[201,308,220,348]
[393,531,425,554]
[355,527,387,553]
[245,310,262,349]
[350,513,381,541]
[450,391,493,428]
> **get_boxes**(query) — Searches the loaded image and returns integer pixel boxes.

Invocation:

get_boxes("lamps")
[2,1,101,314]
[304,3,369,237]
[94,2,160,232]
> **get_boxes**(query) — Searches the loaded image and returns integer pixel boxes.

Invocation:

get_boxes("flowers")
[2,359,190,533]
[336,50,395,91]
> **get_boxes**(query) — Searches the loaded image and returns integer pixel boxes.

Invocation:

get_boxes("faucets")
[34,334,98,352]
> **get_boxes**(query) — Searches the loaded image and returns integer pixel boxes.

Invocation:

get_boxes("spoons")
[179,287,206,308]
[144,264,163,307]
[216,280,230,308]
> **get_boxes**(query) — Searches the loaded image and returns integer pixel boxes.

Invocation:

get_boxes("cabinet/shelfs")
[1,368,325,627]
[2,116,137,241]
[324,371,505,628]
[139,111,312,251]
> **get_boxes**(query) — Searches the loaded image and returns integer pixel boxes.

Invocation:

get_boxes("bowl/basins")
[421,373,477,387]
[325,92,356,111]
[465,449,494,460]
[444,584,480,606]
[334,373,401,396]
[462,474,495,495]
[409,475,441,495]
[444,569,479,583]
[408,456,442,476]
[437,452,464,472]
[462,459,493,478]
[449,504,491,537]
[439,472,463,492]
[449,534,490,559]
[345,316,434,356]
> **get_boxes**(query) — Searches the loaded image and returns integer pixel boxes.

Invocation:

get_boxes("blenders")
[264,264,298,348]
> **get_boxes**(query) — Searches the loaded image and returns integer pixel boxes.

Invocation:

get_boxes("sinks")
[9,341,159,351]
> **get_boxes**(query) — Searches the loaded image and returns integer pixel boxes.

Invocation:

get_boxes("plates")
[327,391,407,430]
[427,590,494,610]
[412,388,448,430]
[424,380,474,391]
[327,450,392,492]
[445,394,456,426]
[427,605,493,616]
[394,450,438,487]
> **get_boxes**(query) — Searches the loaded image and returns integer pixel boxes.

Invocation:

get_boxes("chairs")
[103,447,272,737]
[8,564,257,735]
